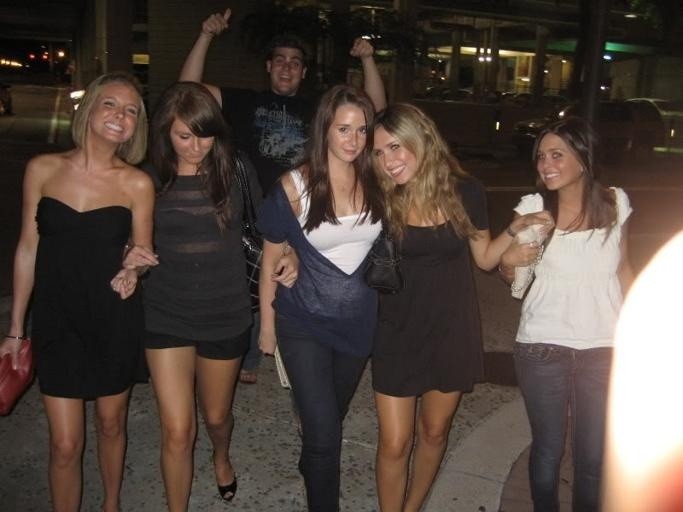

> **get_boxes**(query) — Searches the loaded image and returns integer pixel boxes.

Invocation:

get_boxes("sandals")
[217,476,237,501]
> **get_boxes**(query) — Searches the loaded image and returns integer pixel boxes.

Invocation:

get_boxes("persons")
[498,114,638,512]
[364,99,555,511]
[0,66,17,116]
[255,83,385,511]
[0,71,161,510]
[175,5,387,200]
[121,78,302,511]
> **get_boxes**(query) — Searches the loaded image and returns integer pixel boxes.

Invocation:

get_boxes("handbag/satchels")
[366,229,408,296]
[509,192,558,300]
[0,337,37,418]
[233,156,263,312]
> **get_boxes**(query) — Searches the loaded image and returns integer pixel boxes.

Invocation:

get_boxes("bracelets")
[504,225,517,238]
[3,332,25,341]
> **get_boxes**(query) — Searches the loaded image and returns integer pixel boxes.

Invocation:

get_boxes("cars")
[623,97,682,143]
[509,97,666,162]
[0,79,12,117]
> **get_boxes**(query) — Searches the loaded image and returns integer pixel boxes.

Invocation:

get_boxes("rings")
[529,243,533,249]
